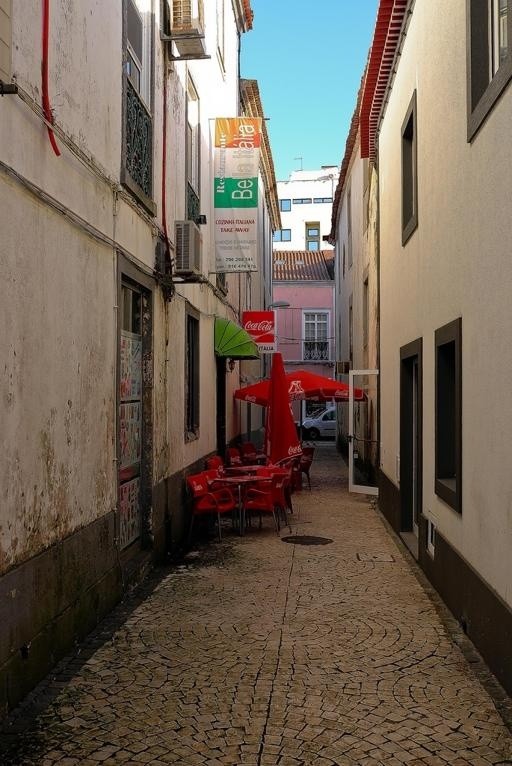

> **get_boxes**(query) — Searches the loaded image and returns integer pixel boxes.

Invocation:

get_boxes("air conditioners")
[174,220,204,279]
[171,0,207,56]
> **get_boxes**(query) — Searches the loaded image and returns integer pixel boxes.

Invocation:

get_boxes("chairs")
[187,441,314,543]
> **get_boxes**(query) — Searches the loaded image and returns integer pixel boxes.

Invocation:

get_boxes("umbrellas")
[265,351,304,469]
[235,369,364,443]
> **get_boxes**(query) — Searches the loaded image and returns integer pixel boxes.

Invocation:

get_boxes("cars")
[297,405,338,442]
